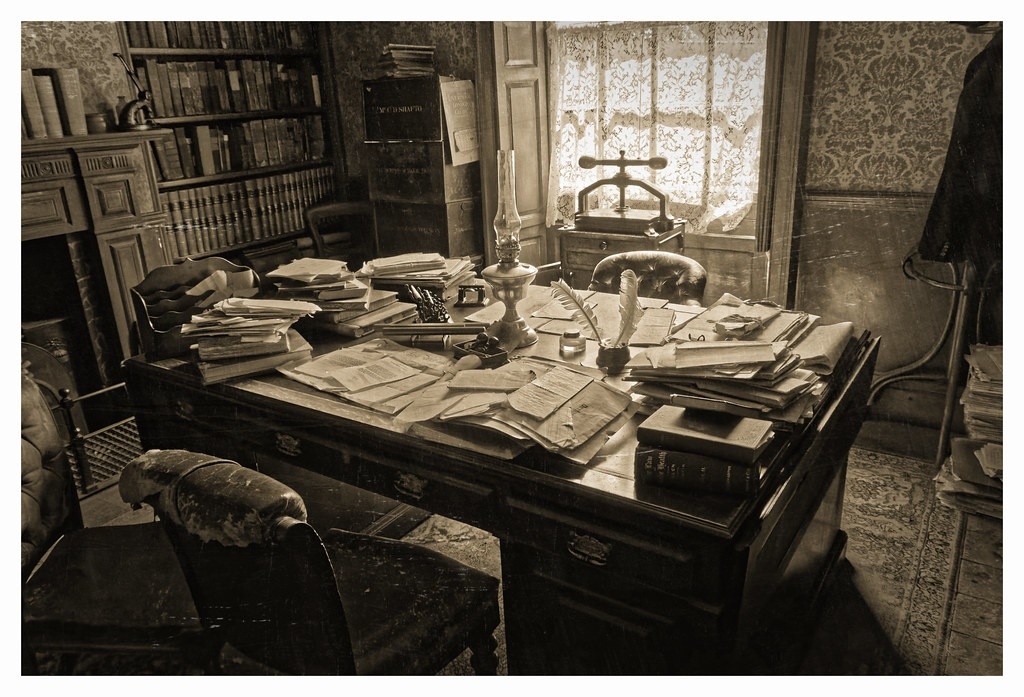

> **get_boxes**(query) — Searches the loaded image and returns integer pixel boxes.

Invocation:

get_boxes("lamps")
[480,150,540,349]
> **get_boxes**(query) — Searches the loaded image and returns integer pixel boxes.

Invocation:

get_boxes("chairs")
[21,367,228,676]
[587,250,707,307]
[301,195,375,262]
[117,449,501,676]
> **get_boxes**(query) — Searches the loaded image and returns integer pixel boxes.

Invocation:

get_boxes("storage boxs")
[360,75,488,279]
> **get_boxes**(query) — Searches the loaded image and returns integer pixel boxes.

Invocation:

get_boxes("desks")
[120,278,882,676]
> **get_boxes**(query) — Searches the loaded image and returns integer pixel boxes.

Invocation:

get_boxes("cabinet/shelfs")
[113,21,352,269]
[556,218,686,290]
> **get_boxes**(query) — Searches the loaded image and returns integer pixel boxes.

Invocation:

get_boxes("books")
[930,343,1003,519]
[21,67,88,139]
[136,59,322,116]
[125,20,321,50]
[158,165,336,258]
[378,43,435,78]
[180,252,854,497]
[150,116,324,182]
